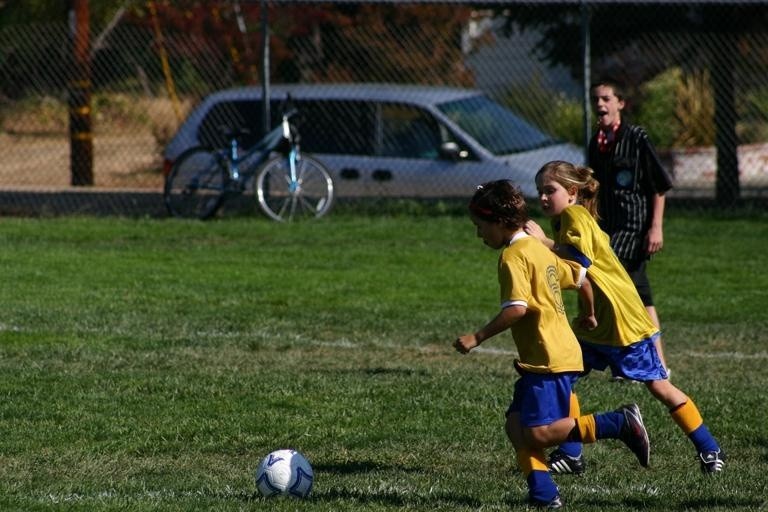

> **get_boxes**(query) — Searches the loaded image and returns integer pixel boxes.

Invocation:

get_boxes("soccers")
[255,449,313,500]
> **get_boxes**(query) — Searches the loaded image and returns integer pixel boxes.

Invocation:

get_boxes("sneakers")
[695,447,725,475]
[614,402,650,466]
[546,454,585,474]
[524,490,562,508]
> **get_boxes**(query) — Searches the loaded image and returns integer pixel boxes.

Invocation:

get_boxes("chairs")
[405,118,434,151]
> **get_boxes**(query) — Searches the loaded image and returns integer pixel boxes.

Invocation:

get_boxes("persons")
[525,161,728,475]
[453,179,650,510]
[586,77,671,383]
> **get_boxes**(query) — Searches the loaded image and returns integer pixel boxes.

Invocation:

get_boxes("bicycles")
[164,93,336,222]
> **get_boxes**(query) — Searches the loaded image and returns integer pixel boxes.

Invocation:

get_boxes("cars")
[163,85,585,199]
